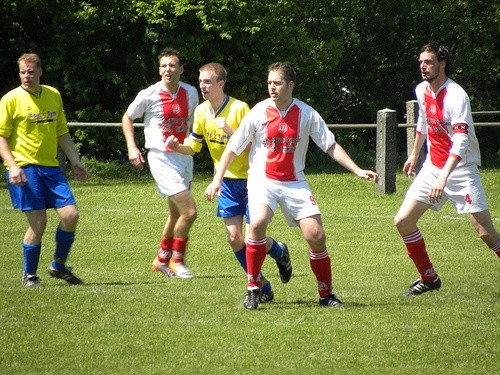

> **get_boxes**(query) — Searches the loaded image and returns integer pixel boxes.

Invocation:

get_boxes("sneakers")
[152,257,176,278]
[244,289,263,310]
[259,289,273,303]
[169,259,194,278]
[48,264,82,284]
[319,293,342,307]
[275,241,292,283]
[404,276,442,297]
[21,272,44,287]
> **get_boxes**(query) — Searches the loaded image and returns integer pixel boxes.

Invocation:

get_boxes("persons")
[164,63,293,305]
[394,41,499,297]
[0,53,89,289]
[121,49,199,278]
[203,63,379,310]
